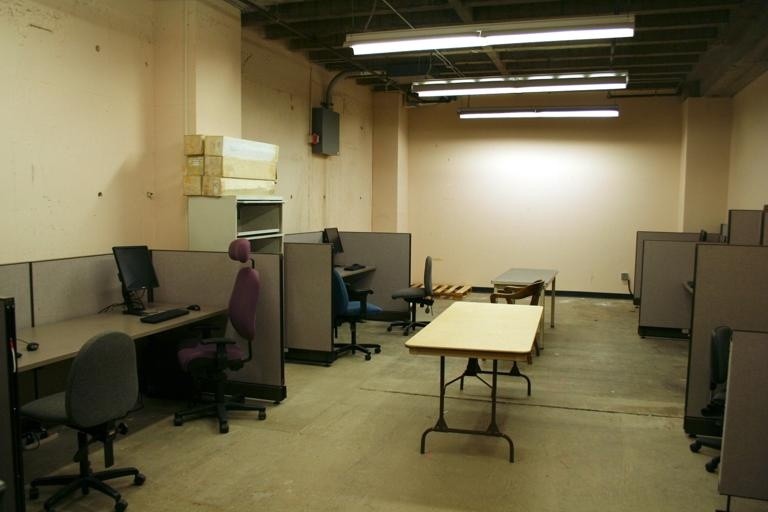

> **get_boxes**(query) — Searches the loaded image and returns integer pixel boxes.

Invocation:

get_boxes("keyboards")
[140,308,189,323]
[343,266,366,271]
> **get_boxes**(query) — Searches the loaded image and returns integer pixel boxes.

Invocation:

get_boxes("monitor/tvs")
[699,230,708,241]
[324,228,344,253]
[112,246,160,310]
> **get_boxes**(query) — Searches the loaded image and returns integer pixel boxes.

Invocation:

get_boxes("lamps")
[412,71,630,97]
[456,105,620,123]
[345,11,636,58]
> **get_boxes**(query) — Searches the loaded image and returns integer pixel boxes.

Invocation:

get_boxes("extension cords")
[23,430,59,449]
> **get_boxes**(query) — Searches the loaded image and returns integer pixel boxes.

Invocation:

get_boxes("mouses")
[352,264,360,266]
[188,305,201,311]
[27,343,39,351]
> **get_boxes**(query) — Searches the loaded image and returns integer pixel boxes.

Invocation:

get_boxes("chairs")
[171,238,267,435]
[335,271,382,359]
[25,329,146,508]
[482,279,543,365]
[689,326,733,470]
[387,255,434,335]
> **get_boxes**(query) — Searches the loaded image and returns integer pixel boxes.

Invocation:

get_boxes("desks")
[405,301,545,465]
[491,267,560,350]
[281,229,412,368]
[2,250,287,511]
